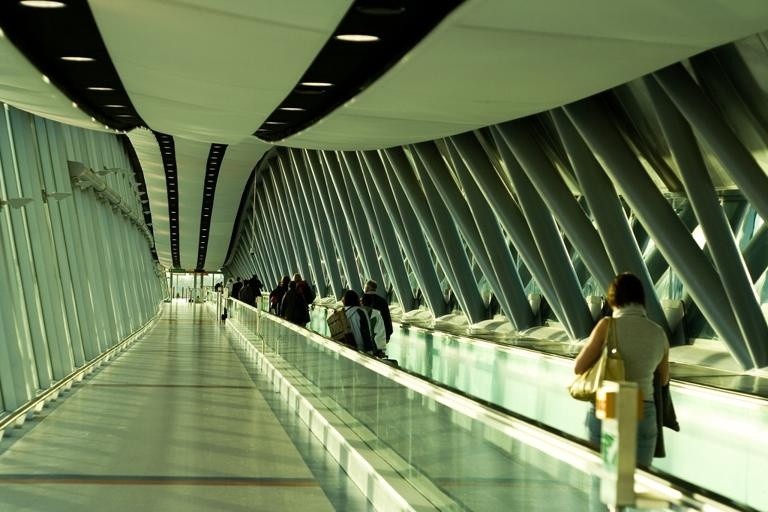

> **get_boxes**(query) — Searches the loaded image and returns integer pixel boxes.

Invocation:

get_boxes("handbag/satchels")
[567,316,627,401]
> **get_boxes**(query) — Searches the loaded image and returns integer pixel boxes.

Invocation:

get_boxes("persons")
[214,272,393,360]
[573,271,673,468]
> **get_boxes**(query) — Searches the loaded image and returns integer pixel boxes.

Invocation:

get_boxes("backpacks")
[326,305,355,346]
